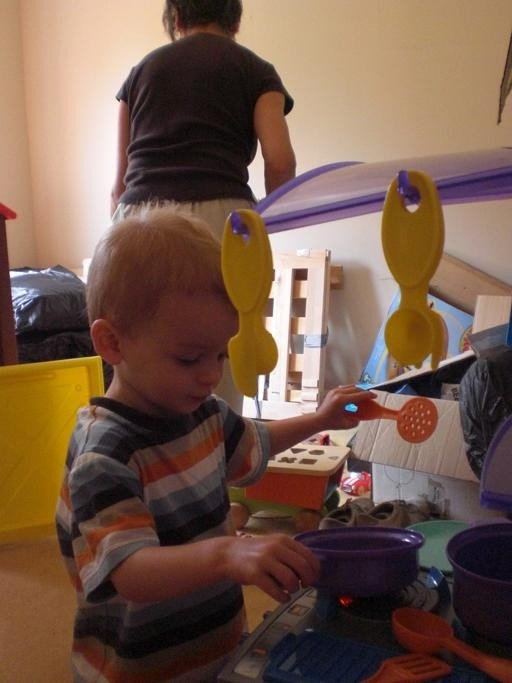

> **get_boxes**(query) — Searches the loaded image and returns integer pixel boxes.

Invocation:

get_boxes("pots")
[291,524,427,600]
[443,522,512,645]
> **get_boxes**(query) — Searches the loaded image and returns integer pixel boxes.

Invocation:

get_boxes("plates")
[405,520,480,570]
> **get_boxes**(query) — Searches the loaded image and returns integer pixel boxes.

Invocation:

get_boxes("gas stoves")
[215,563,512,683]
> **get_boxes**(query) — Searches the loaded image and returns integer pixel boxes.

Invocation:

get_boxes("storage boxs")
[351,340,512,525]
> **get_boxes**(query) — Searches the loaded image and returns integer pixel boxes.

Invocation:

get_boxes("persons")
[107,0,298,414]
[55,201,380,682]
